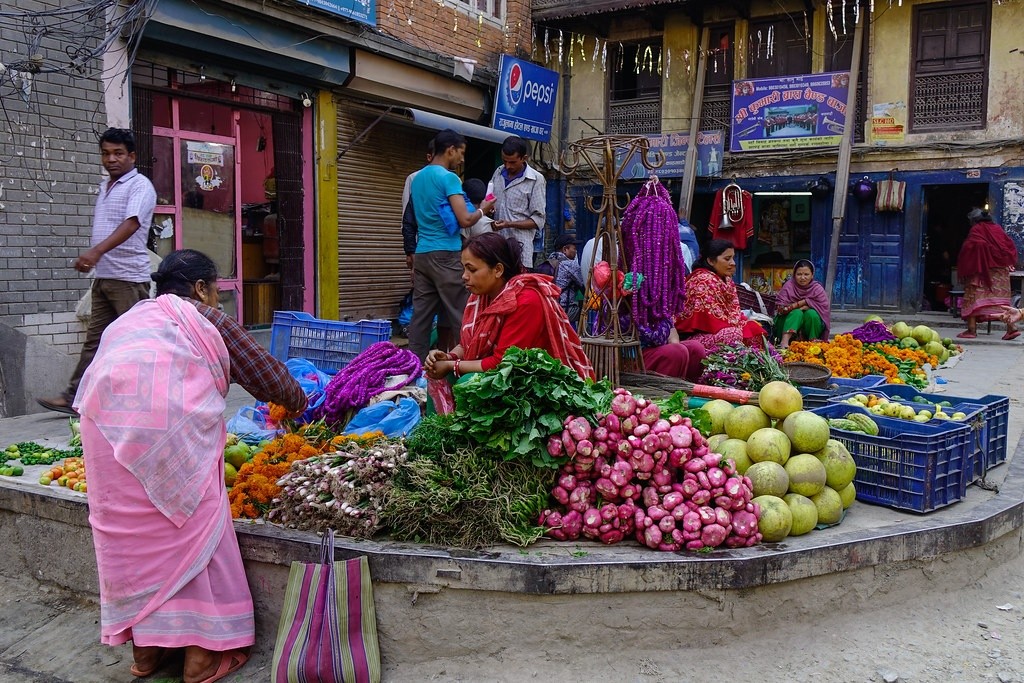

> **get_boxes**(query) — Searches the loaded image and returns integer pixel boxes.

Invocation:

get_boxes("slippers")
[958,331,976,338]
[1002,329,1021,340]
[131,649,176,676]
[191,645,251,683]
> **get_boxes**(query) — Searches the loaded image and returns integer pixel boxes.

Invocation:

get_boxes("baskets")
[778,362,832,390]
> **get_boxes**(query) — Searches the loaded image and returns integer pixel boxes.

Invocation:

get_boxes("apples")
[39,458,88,493]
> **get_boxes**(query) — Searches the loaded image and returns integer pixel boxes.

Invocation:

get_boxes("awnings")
[336,101,519,160]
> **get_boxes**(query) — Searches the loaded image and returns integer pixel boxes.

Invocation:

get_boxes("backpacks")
[534,258,566,279]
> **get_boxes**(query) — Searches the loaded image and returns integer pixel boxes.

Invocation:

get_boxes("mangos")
[222,439,271,492]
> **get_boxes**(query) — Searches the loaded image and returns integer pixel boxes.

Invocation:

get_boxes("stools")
[948,290,965,318]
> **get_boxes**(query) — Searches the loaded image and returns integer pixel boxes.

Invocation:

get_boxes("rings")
[84,264,88,266]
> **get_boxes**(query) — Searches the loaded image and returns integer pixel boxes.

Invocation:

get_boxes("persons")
[424,232,595,381]
[991,305,1024,325]
[400,128,547,361]
[773,259,831,348]
[548,235,585,321]
[765,112,817,136]
[708,145,721,176]
[593,301,705,379]
[73,248,309,681]
[37,127,156,412]
[735,82,752,95]
[833,74,849,88]
[675,240,768,349]
[580,216,622,289]
[957,208,1021,340]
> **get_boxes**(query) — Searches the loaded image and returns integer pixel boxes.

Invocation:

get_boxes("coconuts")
[700,379,856,541]
[863,314,949,365]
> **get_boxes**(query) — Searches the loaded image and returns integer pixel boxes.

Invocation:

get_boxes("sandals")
[36,396,77,414]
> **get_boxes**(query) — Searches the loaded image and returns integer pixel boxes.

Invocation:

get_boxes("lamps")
[300,92,312,107]
[223,71,239,92]
[191,63,207,83]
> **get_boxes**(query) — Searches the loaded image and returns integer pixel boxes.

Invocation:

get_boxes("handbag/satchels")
[76,281,94,320]
[271,527,381,683]
[875,172,906,212]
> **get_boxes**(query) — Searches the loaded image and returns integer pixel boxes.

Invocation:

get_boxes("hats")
[554,236,583,251]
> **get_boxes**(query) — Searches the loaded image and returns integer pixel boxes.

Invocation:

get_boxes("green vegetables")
[385,347,711,547]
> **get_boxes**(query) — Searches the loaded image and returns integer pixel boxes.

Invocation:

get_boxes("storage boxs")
[735,283,777,324]
[796,374,1009,513]
[270,311,392,375]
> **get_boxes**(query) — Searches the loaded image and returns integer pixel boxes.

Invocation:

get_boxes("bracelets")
[454,359,461,378]
[447,352,458,360]
[1019,309,1023,317]
[477,208,483,218]
[797,301,799,307]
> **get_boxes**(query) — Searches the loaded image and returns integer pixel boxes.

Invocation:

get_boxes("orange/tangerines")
[861,337,956,359]
[0,440,83,475]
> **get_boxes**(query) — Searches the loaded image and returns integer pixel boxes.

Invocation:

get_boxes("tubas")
[717,182,744,229]
